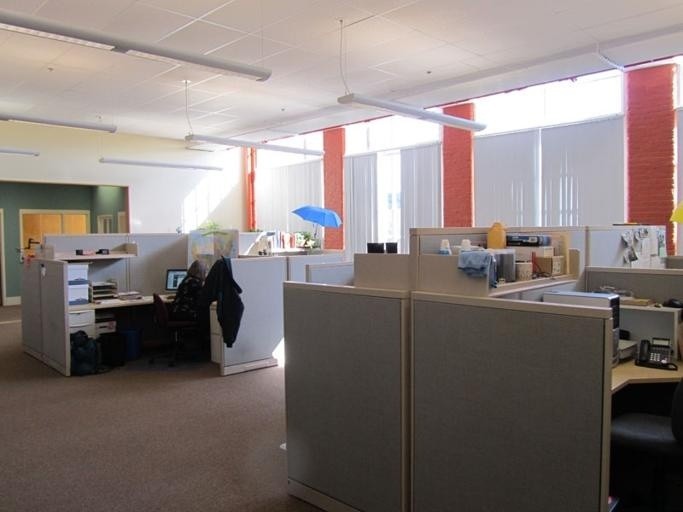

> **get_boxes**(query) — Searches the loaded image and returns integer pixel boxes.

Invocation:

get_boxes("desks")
[19,229,283,377]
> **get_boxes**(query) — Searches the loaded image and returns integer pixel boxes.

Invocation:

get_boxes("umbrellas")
[291,205,342,238]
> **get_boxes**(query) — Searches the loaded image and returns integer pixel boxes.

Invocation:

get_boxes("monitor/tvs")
[163,268,187,293]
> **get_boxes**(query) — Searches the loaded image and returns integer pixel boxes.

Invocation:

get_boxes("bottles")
[439,239,452,255]
[487,222,507,248]
[458,239,472,254]
[516,260,533,281]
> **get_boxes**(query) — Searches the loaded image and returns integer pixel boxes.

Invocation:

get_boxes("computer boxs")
[543,291,620,368]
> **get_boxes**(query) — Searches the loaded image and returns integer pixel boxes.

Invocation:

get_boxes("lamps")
[336,16,487,134]
[182,78,325,157]
[0,22,272,84]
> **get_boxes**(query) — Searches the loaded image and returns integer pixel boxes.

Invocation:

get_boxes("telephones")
[639,337,674,368]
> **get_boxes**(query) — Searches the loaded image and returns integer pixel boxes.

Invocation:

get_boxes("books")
[117,289,143,301]
[261,230,295,250]
